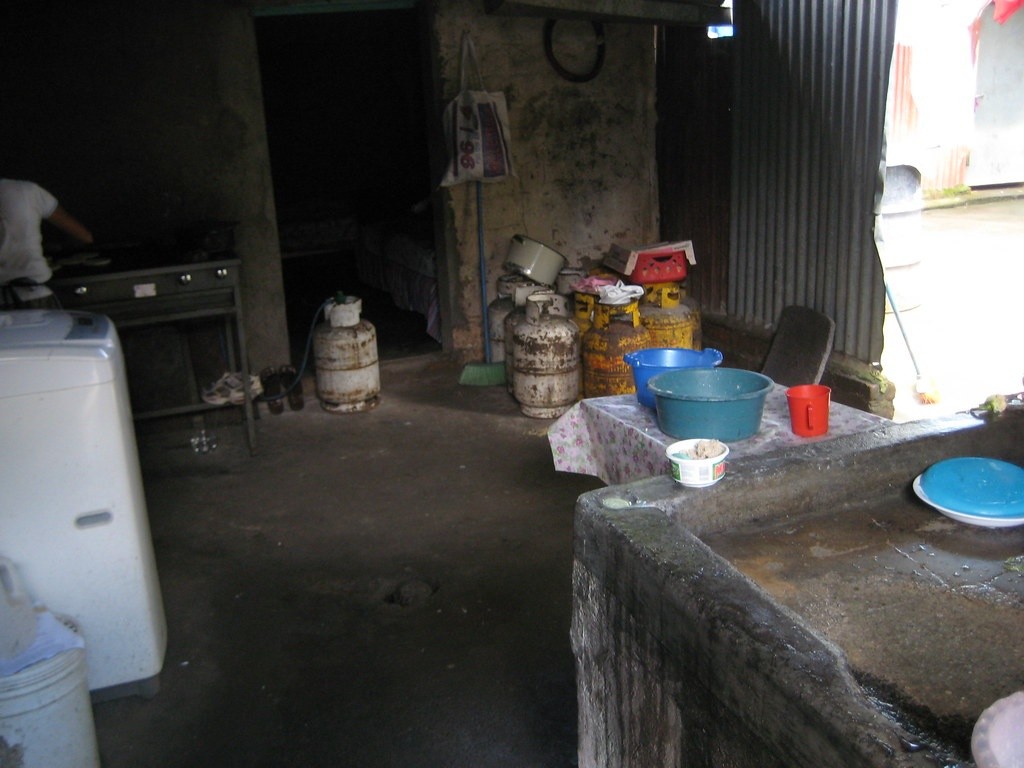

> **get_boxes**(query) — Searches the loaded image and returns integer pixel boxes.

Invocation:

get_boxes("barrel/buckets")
[1,608,105,768]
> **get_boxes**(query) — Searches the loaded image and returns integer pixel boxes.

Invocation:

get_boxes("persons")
[0,177,95,312]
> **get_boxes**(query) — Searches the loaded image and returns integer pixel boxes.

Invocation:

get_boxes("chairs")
[759,304,836,387]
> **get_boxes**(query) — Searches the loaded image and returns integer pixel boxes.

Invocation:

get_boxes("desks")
[547,381,900,484]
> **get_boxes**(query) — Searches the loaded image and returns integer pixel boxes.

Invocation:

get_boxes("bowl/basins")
[646,367,775,442]
[624,347,723,409]
[665,438,729,488]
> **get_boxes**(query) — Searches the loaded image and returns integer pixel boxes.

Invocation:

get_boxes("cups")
[785,384,831,438]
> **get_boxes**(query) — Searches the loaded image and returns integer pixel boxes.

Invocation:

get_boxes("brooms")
[884,283,934,403]
[458,177,506,386]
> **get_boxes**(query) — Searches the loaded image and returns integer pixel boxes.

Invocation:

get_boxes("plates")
[913,473,1024,529]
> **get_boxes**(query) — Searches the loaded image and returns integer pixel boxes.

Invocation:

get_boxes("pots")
[176,218,236,259]
[504,234,567,287]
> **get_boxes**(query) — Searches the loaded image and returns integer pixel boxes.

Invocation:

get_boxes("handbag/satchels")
[438,32,515,188]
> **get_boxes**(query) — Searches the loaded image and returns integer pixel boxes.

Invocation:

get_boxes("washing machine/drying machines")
[2,308,169,694]
